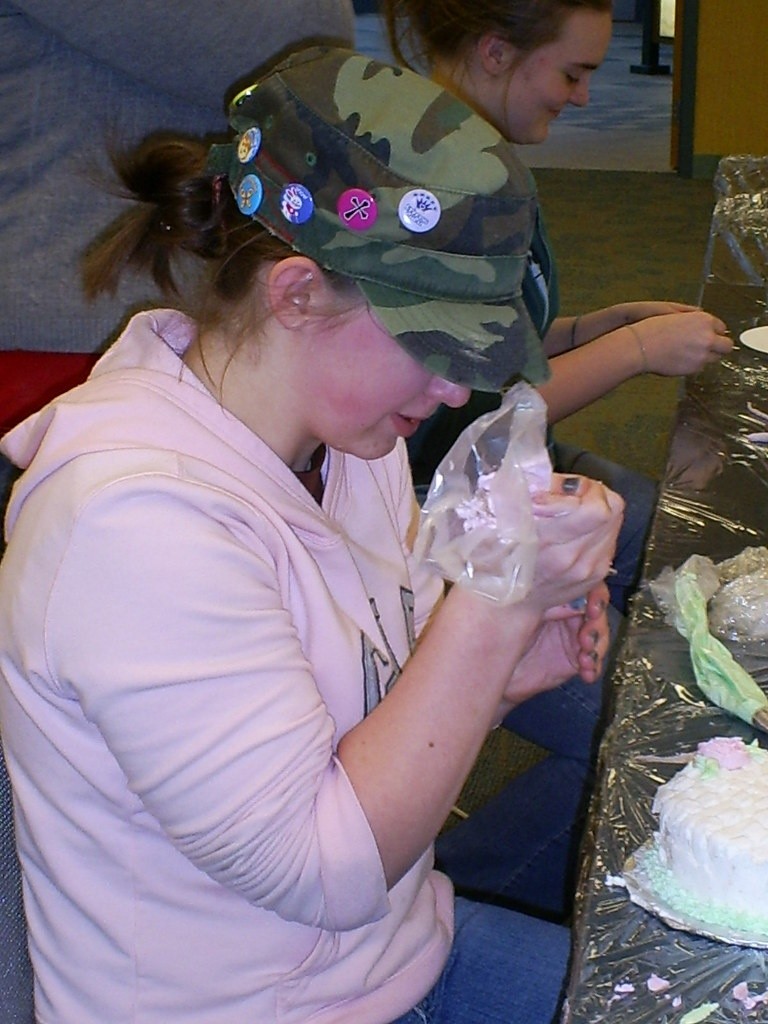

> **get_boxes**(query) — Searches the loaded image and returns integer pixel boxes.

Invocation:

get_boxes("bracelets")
[571,315,583,348]
[625,325,646,376]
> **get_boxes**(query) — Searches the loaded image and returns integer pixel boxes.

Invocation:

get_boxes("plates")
[739,326,768,354]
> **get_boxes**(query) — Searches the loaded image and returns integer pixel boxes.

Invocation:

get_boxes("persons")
[0,46,626,1023]
[390,0,732,921]
[1,0,357,1024]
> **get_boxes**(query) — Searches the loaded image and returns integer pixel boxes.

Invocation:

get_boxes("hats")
[208,44,554,394]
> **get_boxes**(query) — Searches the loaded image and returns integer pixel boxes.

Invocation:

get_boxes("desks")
[562,154,767,1024]
